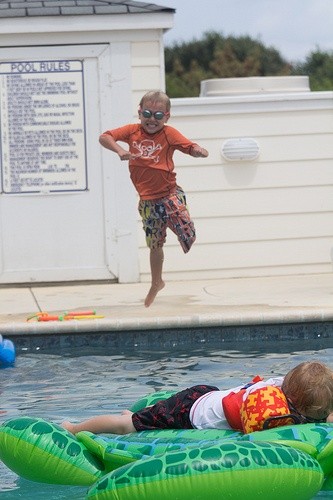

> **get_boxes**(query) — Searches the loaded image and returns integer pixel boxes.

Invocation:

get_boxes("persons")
[98,89,209,310]
[56,358,333,443]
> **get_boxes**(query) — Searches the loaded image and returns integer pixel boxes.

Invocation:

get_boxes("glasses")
[286,402,330,424]
[137,109,171,120]
